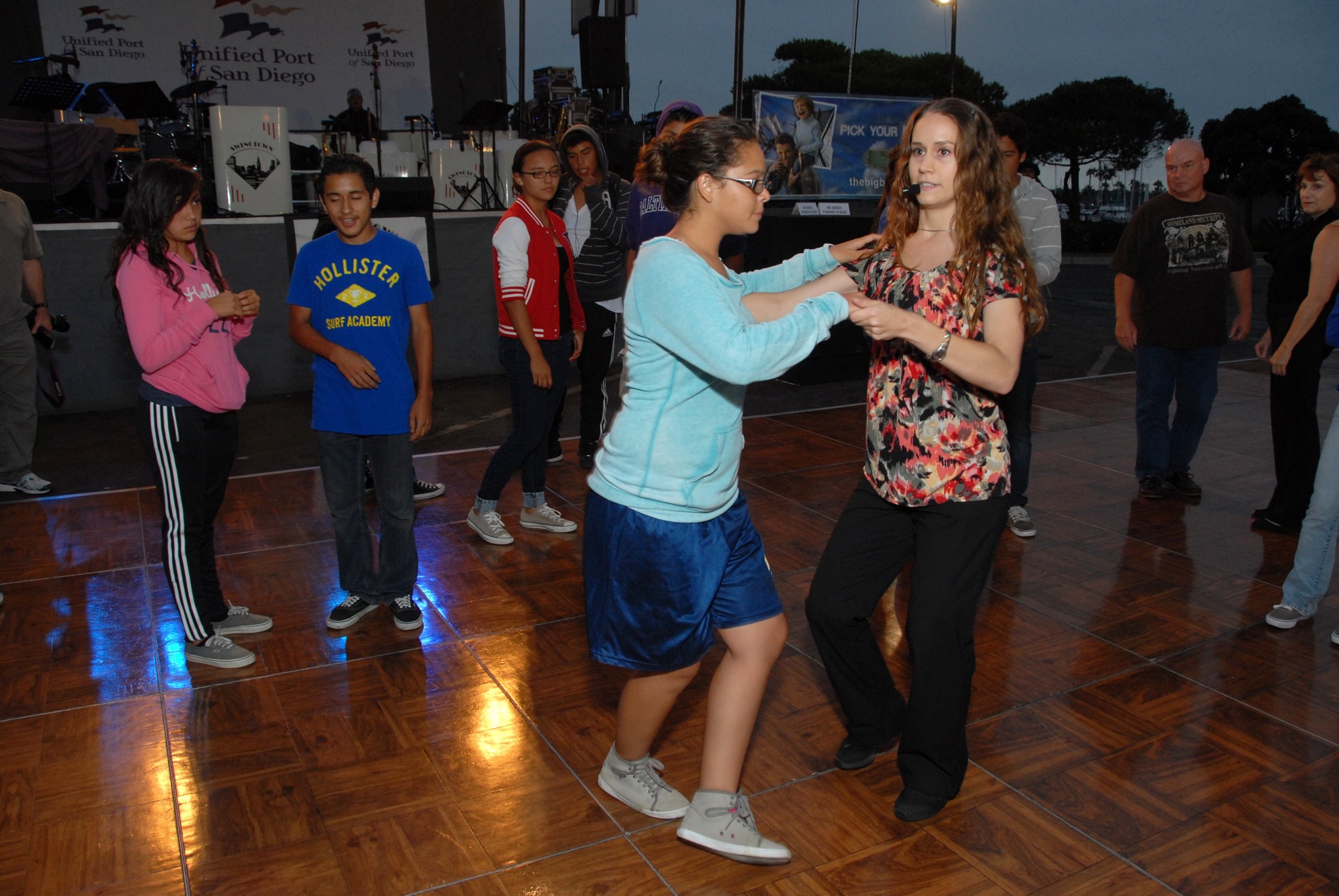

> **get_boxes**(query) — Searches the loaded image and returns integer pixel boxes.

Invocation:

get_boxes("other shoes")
[1251,506,1307,522]
[577,442,598,469]
[543,442,564,463]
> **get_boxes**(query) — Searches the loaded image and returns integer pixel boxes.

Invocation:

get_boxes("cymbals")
[170,80,218,99]
[84,82,120,107]
[72,94,110,114]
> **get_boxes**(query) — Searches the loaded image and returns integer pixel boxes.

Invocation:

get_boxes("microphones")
[902,184,920,194]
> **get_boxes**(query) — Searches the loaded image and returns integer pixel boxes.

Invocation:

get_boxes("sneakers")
[182,624,256,669]
[1265,603,1310,629]
[208,599,273,635]
[0,472,53,495]
[466,506,514,545]
[1138,475,1164,498]
[835,735,892,770]
[1331,627,1339,645]
[1005,506,1037,537]
[410,475,445,500]
[519,501,577,533]
[389,592,423,630]
[361,461,415,495]
[1165,466,1203,497]
[596,741,691,819]
[677,787,791,866]
[893,784,950,821]
[325,591,380,629]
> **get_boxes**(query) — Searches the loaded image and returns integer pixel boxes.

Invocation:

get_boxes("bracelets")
[35,303,49,309]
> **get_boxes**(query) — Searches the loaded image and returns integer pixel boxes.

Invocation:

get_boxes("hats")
[656,100,703,138]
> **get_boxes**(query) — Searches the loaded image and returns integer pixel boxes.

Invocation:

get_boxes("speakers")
[468,98,509,129]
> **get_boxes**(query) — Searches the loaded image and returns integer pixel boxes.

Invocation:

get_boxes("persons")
[990,112,1061,536]
[586,115,882,860]
[467,141,587,544]
[110,159,274,669]
[332,88,376,152]
[741,98,1046,821]
[1251,154,1338,533]
[546,124,632,469]
[1111,139,1257,497]
[764,95,823,195]
[0,189,52,494]
[1018,160,1040,179]
[623,102,744,284]
[285,155,435,630]
[313,212,445,500]
[1266,406,1339,644]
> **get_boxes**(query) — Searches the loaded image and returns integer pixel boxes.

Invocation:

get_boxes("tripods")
[456,131,505,211]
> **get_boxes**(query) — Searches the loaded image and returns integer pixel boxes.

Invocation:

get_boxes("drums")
[118,130,177,179]
[143,97,184,135]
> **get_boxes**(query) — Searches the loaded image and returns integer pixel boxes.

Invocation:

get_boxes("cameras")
[24,309,72,351]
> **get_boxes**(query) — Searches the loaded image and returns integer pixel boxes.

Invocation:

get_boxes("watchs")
[924,330,951,363]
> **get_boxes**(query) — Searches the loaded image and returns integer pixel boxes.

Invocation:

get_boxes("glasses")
[712,172,766,195]
[519,168,563,179]
